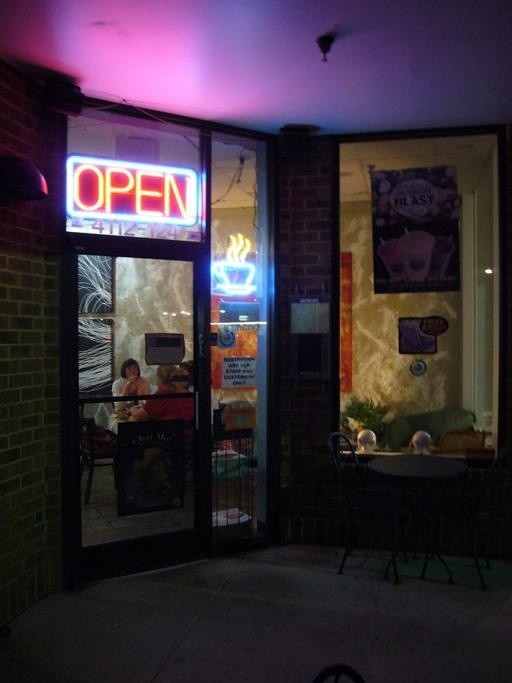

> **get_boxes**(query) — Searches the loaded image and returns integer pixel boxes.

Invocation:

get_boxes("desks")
[355,447,482,558]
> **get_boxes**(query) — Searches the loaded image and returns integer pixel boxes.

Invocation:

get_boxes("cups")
[375,230,456,287]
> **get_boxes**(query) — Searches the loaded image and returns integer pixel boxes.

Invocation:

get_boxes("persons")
[110,357,152,420]
[129,363,196,421]
[178,360,196,377]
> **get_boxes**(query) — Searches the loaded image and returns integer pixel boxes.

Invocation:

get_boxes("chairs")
[327,433,416,578]
[431,446,502,590]
[80,416,126,499]
[438,428,485,449]
